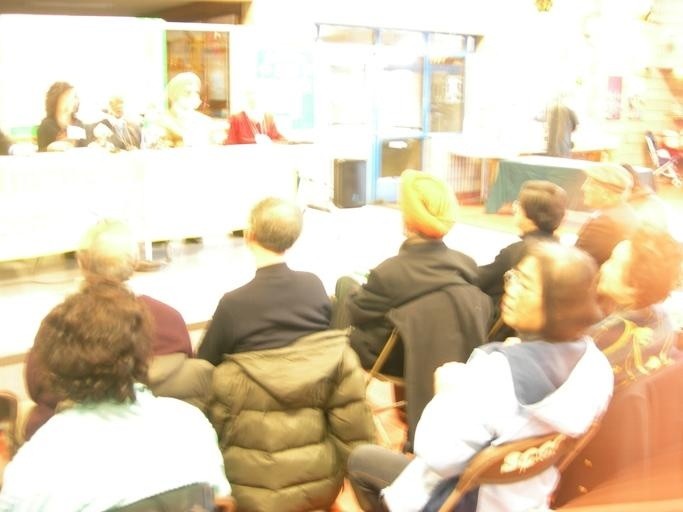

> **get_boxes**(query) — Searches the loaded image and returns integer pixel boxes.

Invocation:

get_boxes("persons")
[546,90,578,158]
[0,197,333,511]
[88,92,141,151]
[333,169,683,512]
[165,70,231,147]
[223,88,286,146]
[37,80,94,151]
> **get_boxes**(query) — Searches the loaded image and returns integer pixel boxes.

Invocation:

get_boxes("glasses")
[504,269,541,297]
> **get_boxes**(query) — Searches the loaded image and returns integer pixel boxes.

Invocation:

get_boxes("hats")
[583,161,631,193]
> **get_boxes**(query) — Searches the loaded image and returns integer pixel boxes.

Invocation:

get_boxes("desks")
[486,155,653,215]
[0,143,296,280]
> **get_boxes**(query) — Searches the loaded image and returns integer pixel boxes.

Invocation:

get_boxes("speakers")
[333,158,365,208]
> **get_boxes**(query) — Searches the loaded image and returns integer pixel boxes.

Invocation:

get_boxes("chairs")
[438,413,607,512]
[364,303,505,450]
[104,481,216,512]
